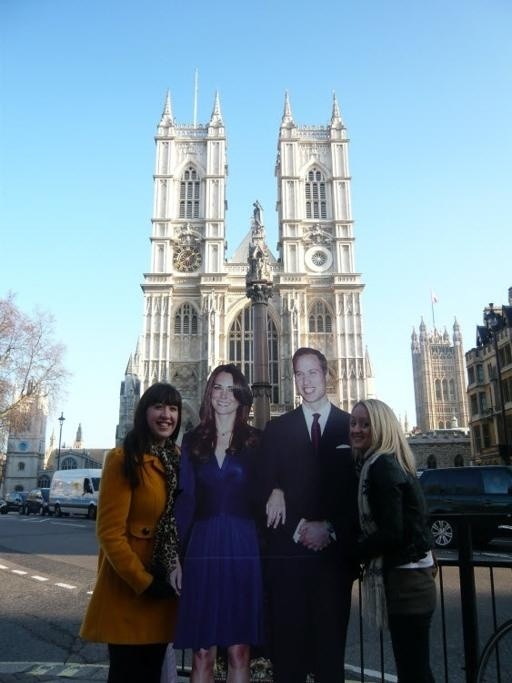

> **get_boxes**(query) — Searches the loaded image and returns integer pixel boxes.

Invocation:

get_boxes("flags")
[431,292,439,305]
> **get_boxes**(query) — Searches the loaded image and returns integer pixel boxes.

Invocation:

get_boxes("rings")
[278,512,282,516]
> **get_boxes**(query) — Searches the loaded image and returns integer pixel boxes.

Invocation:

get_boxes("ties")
[311,412,324,459]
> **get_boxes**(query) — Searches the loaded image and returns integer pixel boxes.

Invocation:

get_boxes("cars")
[0,486,50,515]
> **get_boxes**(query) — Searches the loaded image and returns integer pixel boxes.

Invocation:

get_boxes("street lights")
[56,409,66,470]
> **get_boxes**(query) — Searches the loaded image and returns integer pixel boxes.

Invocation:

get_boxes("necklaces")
[221,432,228,437]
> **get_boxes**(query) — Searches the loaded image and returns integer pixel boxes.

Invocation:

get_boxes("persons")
[77,383,183,683]
[259,347,362,683]
[164,362,287,683]
[348,398,438,683]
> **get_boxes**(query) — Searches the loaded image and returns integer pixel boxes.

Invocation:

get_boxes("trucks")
[46,467,103,520]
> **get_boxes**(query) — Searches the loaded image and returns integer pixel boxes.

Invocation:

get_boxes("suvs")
[417,462,512,550]
